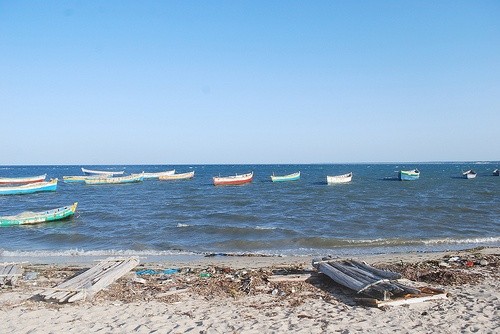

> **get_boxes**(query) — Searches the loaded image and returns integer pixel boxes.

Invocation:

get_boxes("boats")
[270,171,301,182]
[0,177,59,195]
[0,173,47,186]
[31,256,148,304]
[462,169,477,179]
[327,172,352,183]
[0,261,29,290]
[493,169,500,176]
[63,168,195,185]
[311,254,447,308]
[213,171,254,187]
[0,201,78,227]
[398,168,420,181]
